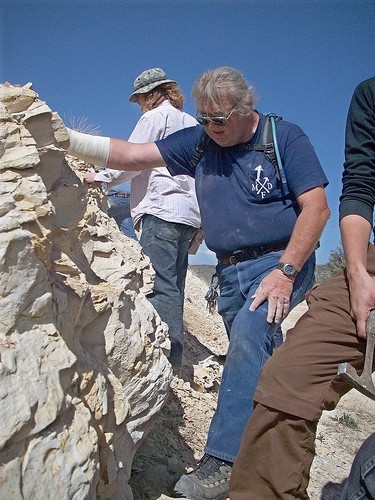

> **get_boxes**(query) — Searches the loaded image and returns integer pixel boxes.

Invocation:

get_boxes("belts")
[216,239,289,266]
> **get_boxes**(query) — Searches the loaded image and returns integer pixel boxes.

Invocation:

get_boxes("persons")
[229,78,375,500]
[84,68,201,364]
[64,68,330,500]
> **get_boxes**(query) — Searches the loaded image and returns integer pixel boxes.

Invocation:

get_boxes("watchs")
[274,263,299,278]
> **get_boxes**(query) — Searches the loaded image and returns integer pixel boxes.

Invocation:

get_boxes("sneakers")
[174,453,233,500]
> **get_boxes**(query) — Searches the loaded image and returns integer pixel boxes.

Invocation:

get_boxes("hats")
[127,67,177,103]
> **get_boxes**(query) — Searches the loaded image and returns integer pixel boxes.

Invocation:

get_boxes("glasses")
[194,104,236,126]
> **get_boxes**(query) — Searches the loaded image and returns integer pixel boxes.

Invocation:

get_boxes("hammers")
[336,275,375,401]
